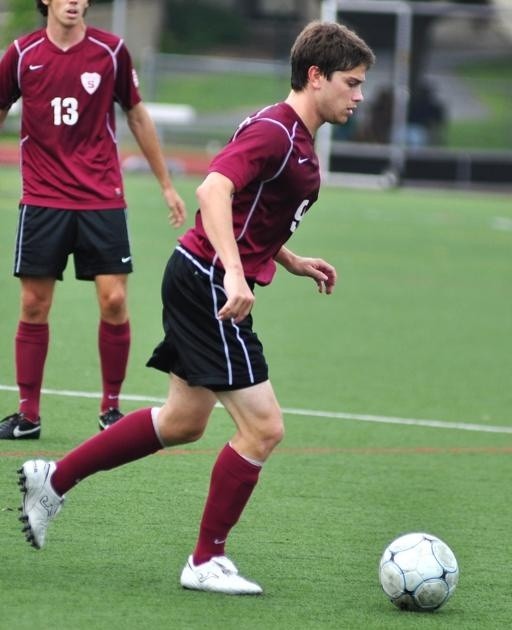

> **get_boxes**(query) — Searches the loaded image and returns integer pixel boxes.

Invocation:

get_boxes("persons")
[0,1,192,444]
[362,46,449,147]
[10,18,380,601]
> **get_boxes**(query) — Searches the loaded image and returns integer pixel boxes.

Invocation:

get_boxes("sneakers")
[0,412,41,439]
[179,553,263,596]
[98,406,124,431]
[15,459,65,551]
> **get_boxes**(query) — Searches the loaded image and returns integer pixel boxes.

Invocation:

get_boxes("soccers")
[377,531,457,615]
